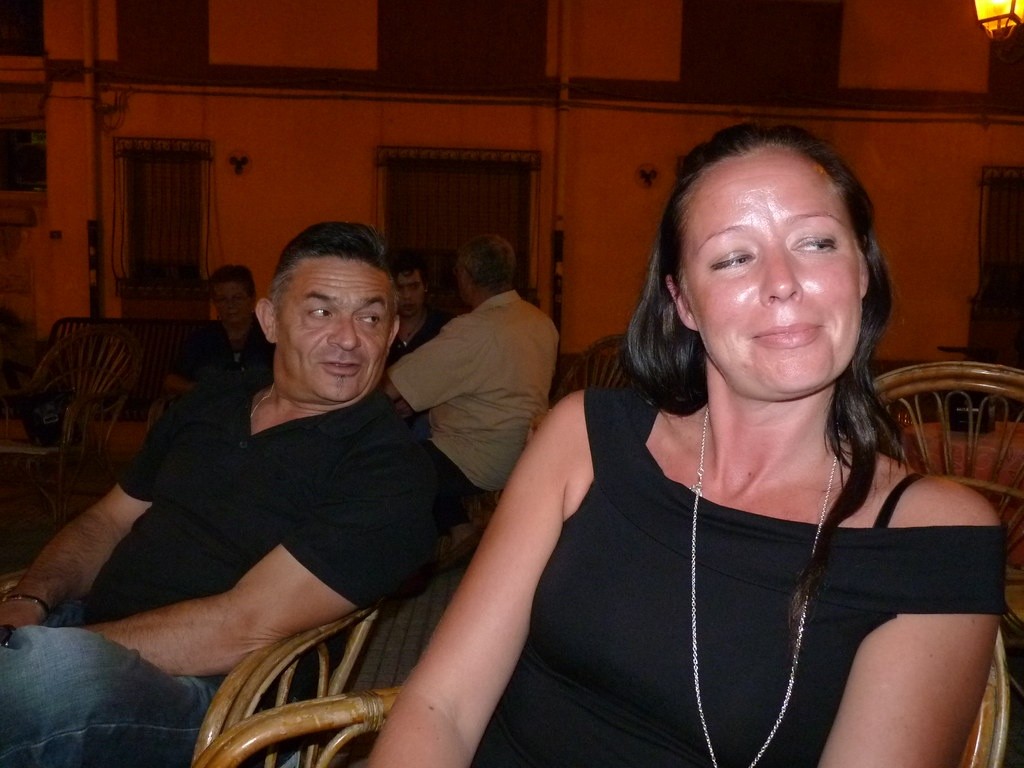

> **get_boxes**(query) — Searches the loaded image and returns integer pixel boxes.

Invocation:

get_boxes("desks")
[904,420,1024,570]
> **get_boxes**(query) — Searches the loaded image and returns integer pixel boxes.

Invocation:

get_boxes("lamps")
[975,0,1024,41]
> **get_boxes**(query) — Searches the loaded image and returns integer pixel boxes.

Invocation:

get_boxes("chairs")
[464,333,629,529]
[845,362,1024,653]
[0,323,145,530]
[0,558,386,768]
[192,621,1015,768]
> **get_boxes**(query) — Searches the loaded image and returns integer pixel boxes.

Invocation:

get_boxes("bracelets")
[2,594,50,623]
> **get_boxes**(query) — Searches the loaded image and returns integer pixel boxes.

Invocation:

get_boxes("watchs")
[0,624,16,647]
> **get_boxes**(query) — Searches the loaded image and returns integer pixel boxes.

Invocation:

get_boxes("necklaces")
[250,382,274,417]
[690,408,838,768]
[398,312,424,347]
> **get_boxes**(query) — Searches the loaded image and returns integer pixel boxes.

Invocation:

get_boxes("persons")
[162,264,274,415]
[0,219,441,768]
[365,123,1011,768]
[378,233,560,566]
[379,246,456,450]
